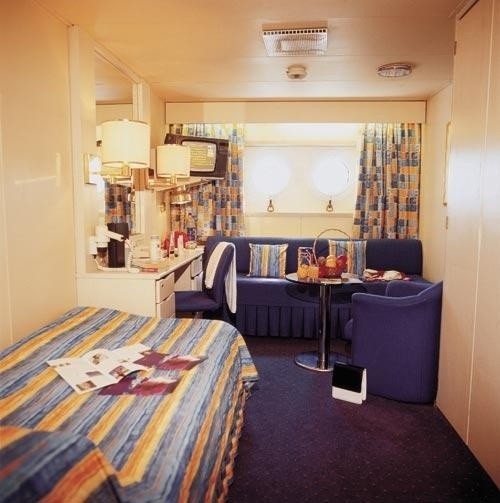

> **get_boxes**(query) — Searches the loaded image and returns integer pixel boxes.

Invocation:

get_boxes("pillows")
[329,240,368,278]
[0,426,129,503]
[245,242,288,278]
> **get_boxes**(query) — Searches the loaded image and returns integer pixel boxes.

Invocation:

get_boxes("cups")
[297,265,320,279]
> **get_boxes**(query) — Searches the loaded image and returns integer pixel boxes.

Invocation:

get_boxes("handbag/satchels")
[331,362,367,405]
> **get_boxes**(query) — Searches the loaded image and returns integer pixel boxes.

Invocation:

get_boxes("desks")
[284,273,363,372]
[76,246,204,320]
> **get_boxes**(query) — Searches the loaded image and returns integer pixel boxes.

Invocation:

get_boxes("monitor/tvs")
[164,134,229,178]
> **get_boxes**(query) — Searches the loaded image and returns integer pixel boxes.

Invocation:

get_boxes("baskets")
[313,228,353,278]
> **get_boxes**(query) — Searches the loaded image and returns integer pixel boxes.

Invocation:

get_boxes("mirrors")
[92,47,145,242]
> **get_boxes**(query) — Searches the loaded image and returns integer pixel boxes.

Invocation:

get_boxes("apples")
[326,256,347,267]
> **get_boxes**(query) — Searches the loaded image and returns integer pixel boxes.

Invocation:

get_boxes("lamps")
[145,144,190,189]
[84,119,151,185]
[262,29,328,57]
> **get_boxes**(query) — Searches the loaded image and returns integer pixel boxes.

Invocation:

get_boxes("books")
[46,349,148,395]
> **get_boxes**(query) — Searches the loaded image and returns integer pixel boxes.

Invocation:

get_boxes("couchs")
[206,237,422,339]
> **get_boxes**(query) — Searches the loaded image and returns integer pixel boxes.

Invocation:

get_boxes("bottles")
[150,235,161,261]
[186,213,196,241]
[300,253,310,267]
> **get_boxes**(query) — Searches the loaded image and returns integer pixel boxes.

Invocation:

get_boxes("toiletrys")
[166,231,185,263]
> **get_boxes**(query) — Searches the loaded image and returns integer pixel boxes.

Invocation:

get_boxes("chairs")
[174,241,234,319]
[344,280,443,404]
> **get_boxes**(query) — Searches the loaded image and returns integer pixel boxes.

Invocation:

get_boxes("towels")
[205,242,237,314]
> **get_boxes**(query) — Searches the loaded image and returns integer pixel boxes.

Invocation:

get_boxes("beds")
[0,306,261,503]
